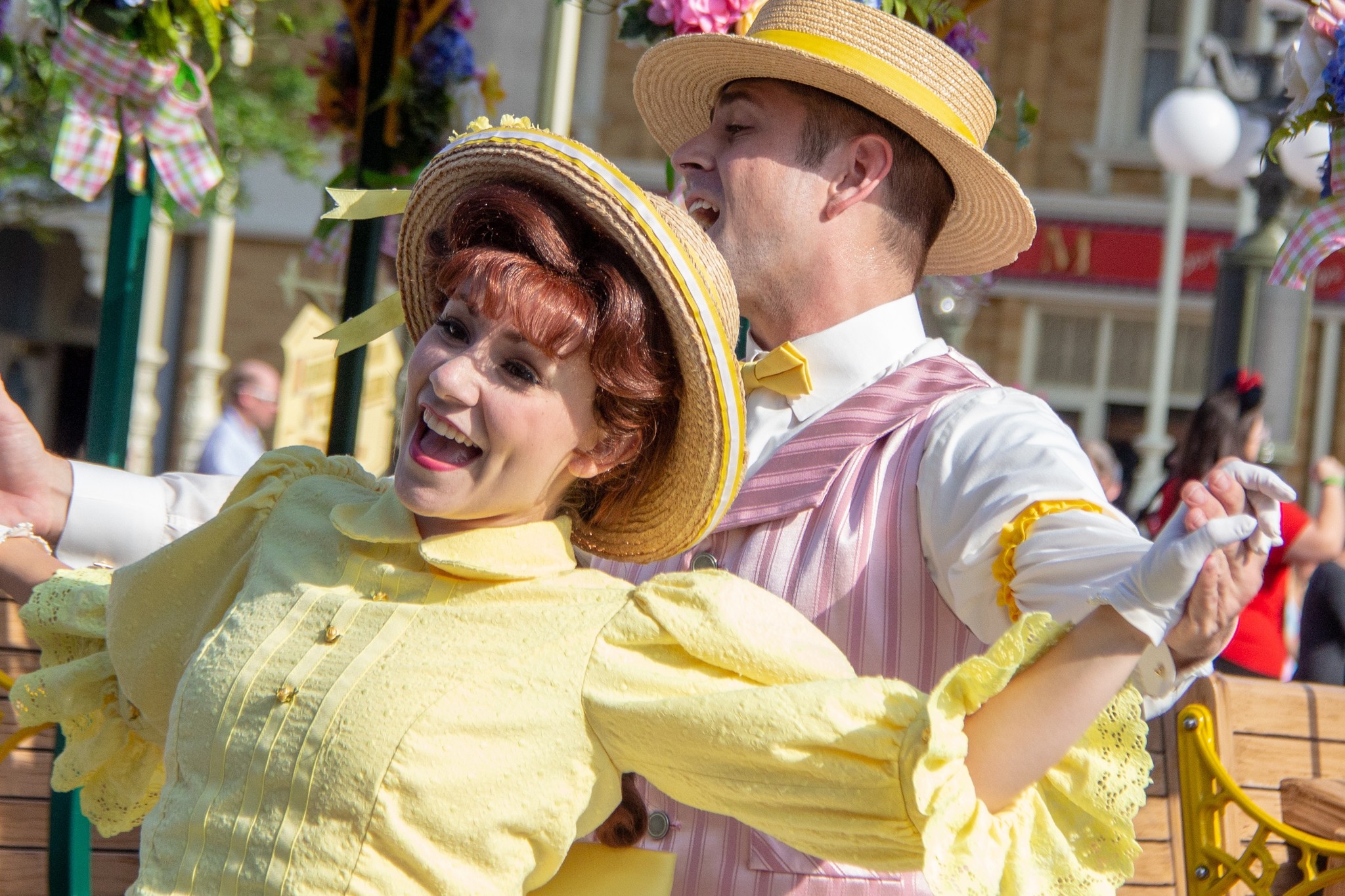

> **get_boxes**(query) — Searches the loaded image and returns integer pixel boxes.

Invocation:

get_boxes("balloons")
[1150,84,1331,191]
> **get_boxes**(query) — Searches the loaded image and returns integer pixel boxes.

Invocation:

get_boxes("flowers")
[576,0,1036,151]
[0,1,321,222]
[277,0,505,172]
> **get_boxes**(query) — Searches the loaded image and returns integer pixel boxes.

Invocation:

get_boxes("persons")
[0,0,1270,895]
[1141,378,1345,681]
[0,108,1296,895]
[199,358,281,476]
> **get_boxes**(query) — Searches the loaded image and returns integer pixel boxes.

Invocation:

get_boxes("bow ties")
[739,340,813,396]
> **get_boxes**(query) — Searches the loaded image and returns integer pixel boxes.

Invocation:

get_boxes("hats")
[313,113,749,564]
[634,0,1037,276]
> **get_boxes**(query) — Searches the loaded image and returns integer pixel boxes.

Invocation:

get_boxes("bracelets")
[1320,477,1344,488]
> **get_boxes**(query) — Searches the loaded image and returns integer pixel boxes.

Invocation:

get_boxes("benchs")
[1178,674,1345,896]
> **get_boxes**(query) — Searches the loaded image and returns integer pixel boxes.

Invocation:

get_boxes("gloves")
[1102,461,1298,645]
